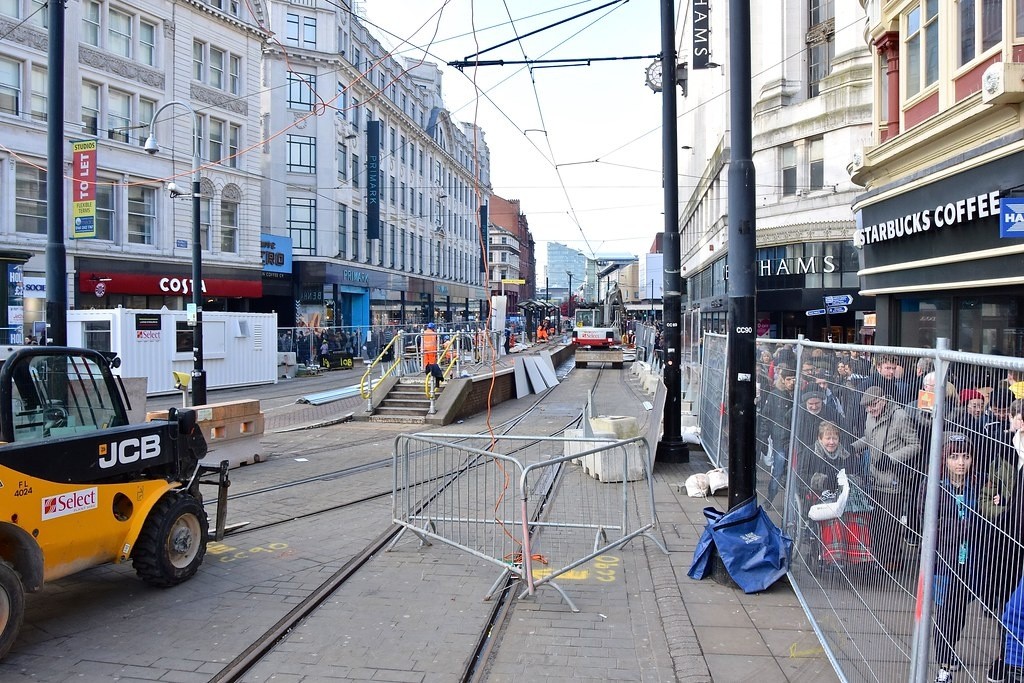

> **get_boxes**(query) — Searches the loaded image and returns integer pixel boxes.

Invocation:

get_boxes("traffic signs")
[805,295,854,316]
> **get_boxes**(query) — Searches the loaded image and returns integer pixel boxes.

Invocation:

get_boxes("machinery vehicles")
[572,308,624,368]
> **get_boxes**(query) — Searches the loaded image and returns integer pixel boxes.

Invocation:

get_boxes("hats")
[960,389,984,406]
[941,431,973,460]
[860,386,884,405]
[801,383,825,403]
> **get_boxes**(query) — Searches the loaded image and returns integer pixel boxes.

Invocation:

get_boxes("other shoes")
[903,537,919,547]
[885,562,905,573]
[764,499,772,510]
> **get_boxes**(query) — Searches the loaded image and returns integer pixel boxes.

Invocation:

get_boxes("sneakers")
[987,661,1008,683]
[935,667,952,683]
[950,654,960,671]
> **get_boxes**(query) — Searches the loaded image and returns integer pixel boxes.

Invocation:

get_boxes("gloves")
[823,477,838,492]
[875,456,892,471]
[845,445,856,454]
[761,441,768,456]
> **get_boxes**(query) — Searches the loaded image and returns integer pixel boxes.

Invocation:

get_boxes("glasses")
[868,399,881,408]
[924,383,935,390]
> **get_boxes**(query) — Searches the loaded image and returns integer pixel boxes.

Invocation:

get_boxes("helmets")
[545,316,550,320]
[443,335,449,340]
[428,323,435,328]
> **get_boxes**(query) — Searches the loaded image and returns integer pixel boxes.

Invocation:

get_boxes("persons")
[621,318,665,375]
[750,335,1024,683]
[505,318,536,332]
[24,328,46,346]
[539,315,557,343]
[278,319,490,365]
[581,316,592,327]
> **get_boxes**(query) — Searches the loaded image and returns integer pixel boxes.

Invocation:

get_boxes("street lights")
[566,271,573,318]
[144,101,207,406]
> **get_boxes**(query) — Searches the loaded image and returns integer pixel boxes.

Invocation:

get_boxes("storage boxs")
[582,439,643,483]
[588,416,638,440]
[563,429,618,462]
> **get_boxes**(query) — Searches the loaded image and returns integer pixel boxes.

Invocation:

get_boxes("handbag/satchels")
[808,469,848,522]
[892,461,925,487]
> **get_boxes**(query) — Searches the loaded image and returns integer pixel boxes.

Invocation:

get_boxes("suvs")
[0,346,231,661]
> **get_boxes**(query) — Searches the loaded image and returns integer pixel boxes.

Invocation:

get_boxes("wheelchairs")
[783,473,878,582]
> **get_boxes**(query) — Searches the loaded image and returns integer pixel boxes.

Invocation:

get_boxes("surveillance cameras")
[144,136,159,154]
[168,183,185,195]
